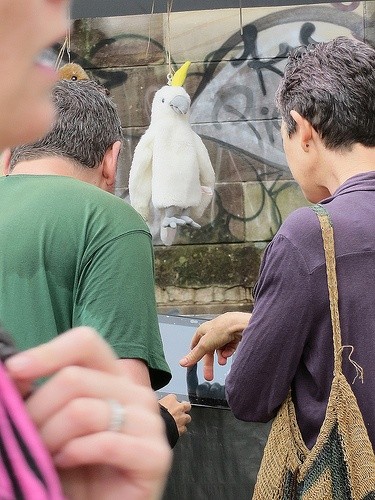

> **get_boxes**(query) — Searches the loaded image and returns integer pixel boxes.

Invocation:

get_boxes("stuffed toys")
[127,60,216,234]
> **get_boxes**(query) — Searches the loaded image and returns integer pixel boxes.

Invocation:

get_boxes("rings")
[106,399,126,434]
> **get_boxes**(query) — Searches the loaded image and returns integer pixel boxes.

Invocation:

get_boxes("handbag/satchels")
[251,373,375,500]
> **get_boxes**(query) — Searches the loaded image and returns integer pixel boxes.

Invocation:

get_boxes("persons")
[0,78,191,450]
[0,0,176,500]
[178,32,375,498]
